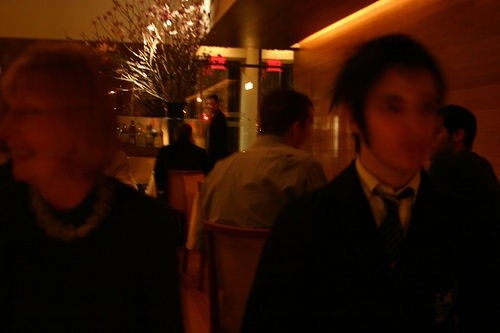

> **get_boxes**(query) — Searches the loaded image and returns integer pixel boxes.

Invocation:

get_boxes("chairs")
[169,169,274,333]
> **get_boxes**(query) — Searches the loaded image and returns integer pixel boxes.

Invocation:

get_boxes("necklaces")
[32,181,108,238]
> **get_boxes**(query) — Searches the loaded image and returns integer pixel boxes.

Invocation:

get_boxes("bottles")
[116,121,155,148]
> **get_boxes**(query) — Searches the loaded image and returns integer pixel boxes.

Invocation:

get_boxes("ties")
[373,187,415,240]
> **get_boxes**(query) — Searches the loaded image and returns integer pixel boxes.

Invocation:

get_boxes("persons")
[204,95,226,175]
[155,123,206,191]
[0,44,184,333]
[202,91,325,227]
[435,105,500,187]
[240,33,500,333]
